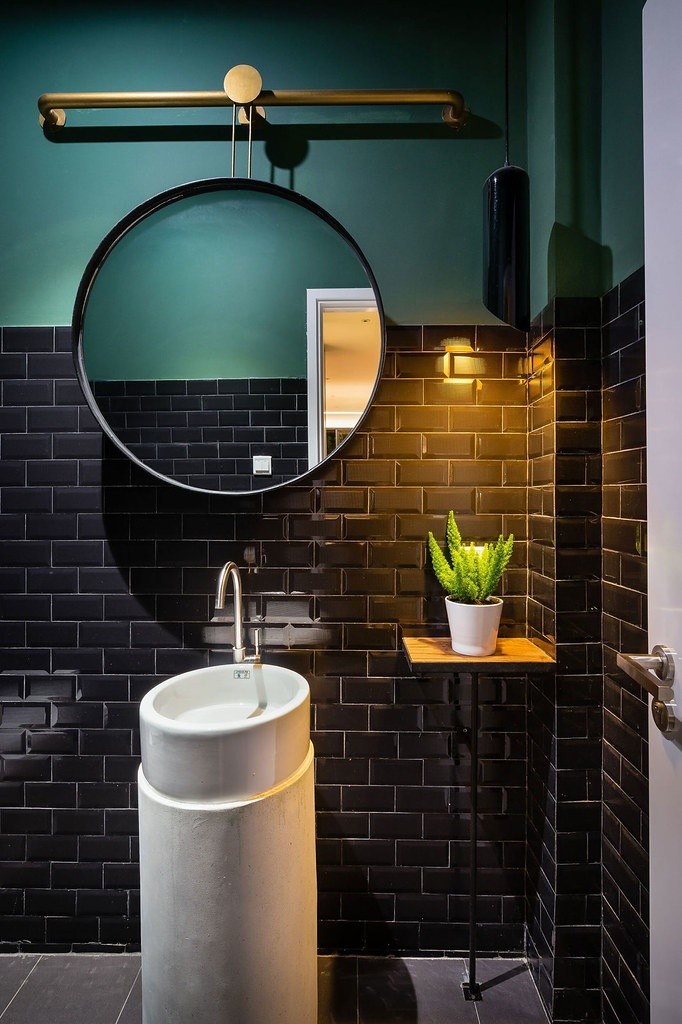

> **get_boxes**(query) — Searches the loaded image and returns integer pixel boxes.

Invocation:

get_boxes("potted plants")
[428,511,513,657]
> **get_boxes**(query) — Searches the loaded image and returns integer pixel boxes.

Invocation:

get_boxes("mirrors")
[70,178,387,500]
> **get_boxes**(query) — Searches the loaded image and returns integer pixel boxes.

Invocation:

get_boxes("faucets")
[213,560,246,664]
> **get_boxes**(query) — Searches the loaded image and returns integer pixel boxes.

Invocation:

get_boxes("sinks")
[138,662,313,805]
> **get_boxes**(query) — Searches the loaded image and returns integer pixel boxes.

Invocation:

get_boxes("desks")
[401,636,555,993]
